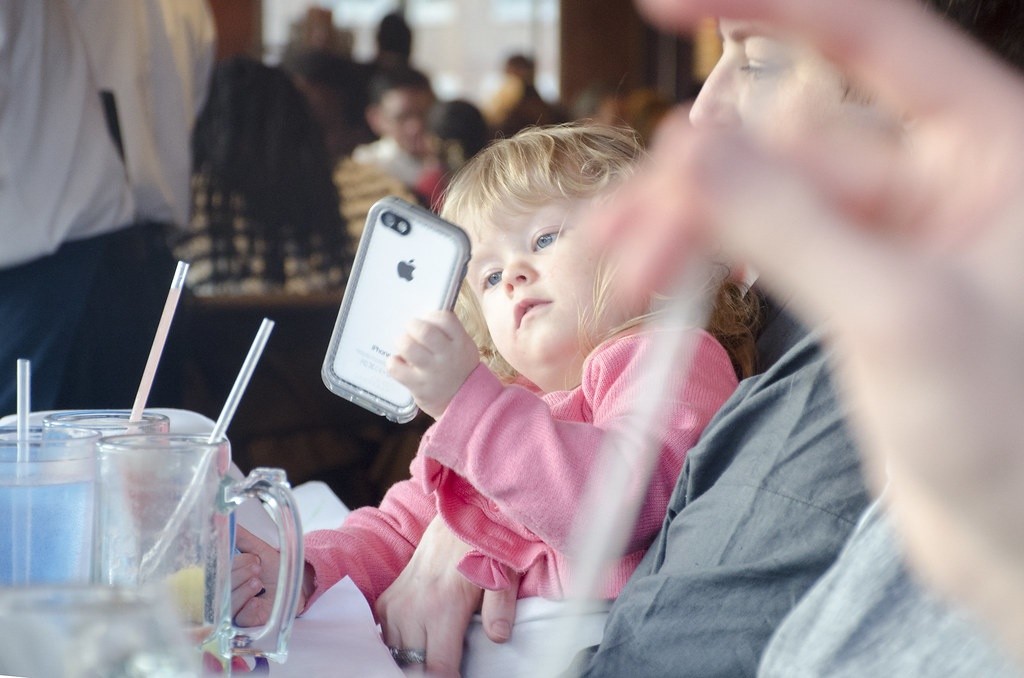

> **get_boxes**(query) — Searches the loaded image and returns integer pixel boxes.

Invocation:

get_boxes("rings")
[389,647,404,665]
[401,649,425,664]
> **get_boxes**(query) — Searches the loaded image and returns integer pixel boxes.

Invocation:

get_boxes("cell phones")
[321,194,472,424]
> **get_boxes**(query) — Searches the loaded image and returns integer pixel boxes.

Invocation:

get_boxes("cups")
[0,408,306,678]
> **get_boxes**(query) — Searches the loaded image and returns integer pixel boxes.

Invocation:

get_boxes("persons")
[0,0,565,508]
[230,123,761,678]
[378,0,1024,678]
